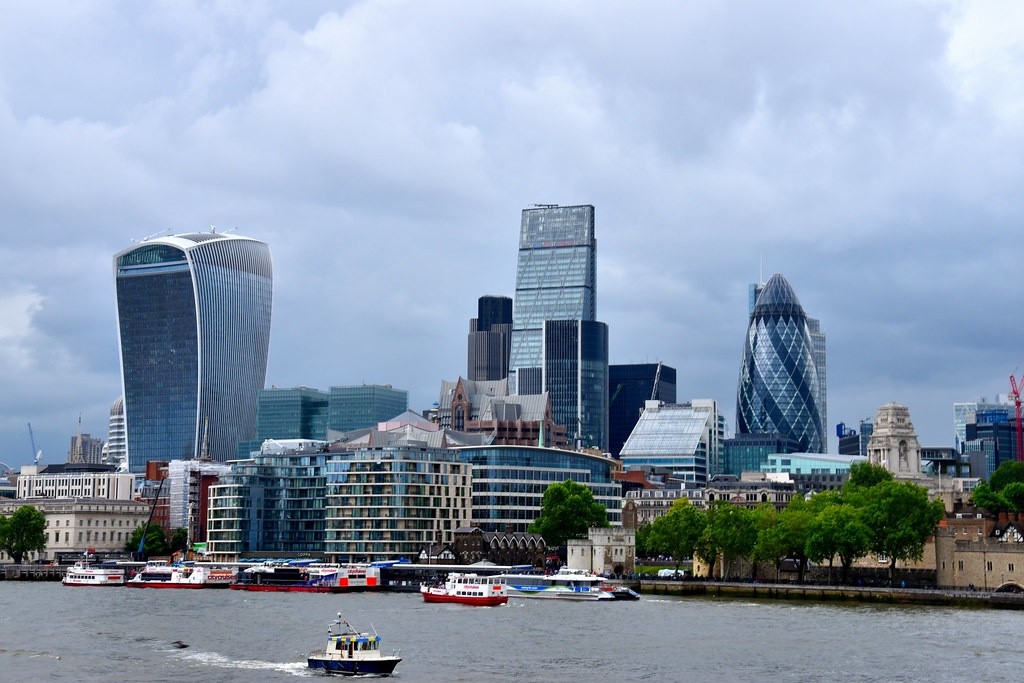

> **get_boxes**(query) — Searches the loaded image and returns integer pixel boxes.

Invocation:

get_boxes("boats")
[480,569,615,601]
[307,612,402,677]
[126,566,234,589]
[229,561,380,594]
[61,560,125,586]
[419,571,509,606]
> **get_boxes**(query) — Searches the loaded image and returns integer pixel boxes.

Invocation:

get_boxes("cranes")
[1008,368,1024,461]
